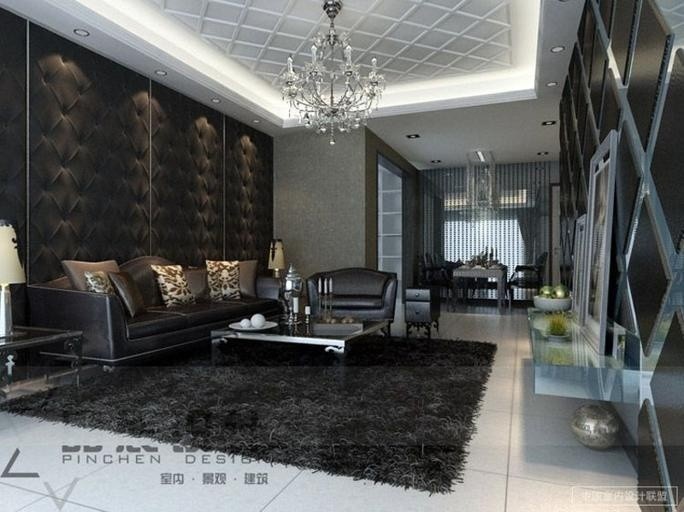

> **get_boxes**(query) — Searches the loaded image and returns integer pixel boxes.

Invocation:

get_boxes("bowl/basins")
[533,295,572,312]
[459,252,500,270]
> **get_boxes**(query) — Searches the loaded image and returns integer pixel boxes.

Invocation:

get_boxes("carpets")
[1,334,499,500]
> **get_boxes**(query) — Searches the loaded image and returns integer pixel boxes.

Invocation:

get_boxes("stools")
[406,286,440,338]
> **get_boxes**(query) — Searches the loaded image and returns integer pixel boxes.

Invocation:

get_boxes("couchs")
[28,255,284,371]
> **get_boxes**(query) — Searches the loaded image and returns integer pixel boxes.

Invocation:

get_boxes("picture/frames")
[571,211,587,329]
[580,122,621,358]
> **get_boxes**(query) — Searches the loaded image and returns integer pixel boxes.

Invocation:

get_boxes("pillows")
[83,270,115,296]
[204,259,240,303]
[61,258,120,293]
[108,270,148,318]
[150,264,196,308]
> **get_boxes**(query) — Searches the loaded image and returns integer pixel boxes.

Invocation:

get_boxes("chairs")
[305,267,398,338]
[421,252,458,312]
[507,251,548,314]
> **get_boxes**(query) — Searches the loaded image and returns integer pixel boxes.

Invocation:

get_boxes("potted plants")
[542,311,570,343]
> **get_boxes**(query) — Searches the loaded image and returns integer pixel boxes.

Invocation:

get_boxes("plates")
[228,321,279,331]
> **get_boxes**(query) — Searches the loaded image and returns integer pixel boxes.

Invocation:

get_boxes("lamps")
[267,238,285,280]
[273,1,388,148]
[0,219,26,337]
